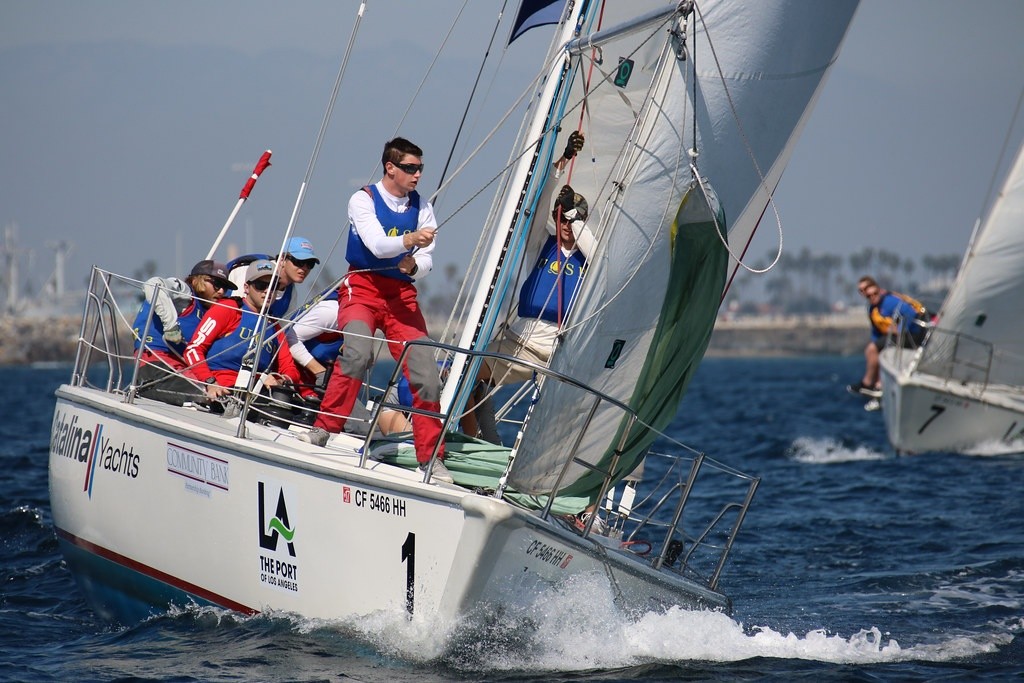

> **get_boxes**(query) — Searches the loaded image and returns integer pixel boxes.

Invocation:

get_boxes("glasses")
[867,291,879,299]
[860,286,869,292]
[249,280,279,291]
[203,278,228,292]
[556,212,573,223]
[390,161,424,174]
[285,256,315,269]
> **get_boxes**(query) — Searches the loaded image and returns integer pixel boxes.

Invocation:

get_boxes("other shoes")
[859,386,884,397]
[864,399,881,411]
[848,382,873,395]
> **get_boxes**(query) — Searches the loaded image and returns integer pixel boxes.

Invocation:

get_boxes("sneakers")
[299,427,331,447]
[416,457,454,483]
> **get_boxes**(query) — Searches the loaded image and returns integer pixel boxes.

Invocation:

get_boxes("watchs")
[203,375,217,387]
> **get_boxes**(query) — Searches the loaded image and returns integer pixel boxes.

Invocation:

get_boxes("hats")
[245,259,282,283]
[554,193,588,220]
[191,260,238,290]
[284,236,320,265]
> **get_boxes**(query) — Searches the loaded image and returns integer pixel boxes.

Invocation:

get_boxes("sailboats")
[842,137,1024,458]
[51,0,860,670]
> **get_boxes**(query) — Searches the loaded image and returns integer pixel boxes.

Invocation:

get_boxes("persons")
[459,129,600,439]
[846,274,930,413]
[131,236,345,424]
[306,136,486,485]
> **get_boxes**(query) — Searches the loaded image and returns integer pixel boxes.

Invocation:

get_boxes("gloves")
[164,326,182,345]
[558,184,575,211]
[563,131,585,160]
